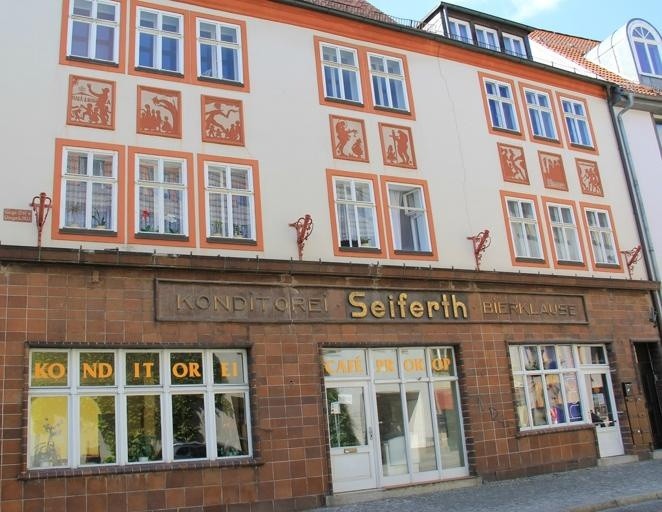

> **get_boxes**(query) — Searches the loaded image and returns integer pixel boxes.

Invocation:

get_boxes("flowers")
[142,209,150,229]
[164,213,177,233]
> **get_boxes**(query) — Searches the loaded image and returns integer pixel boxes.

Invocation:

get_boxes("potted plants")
[92,208,108,229]
[361,237,373,248]
[233,224,244,238]
[212,220,222,236]
